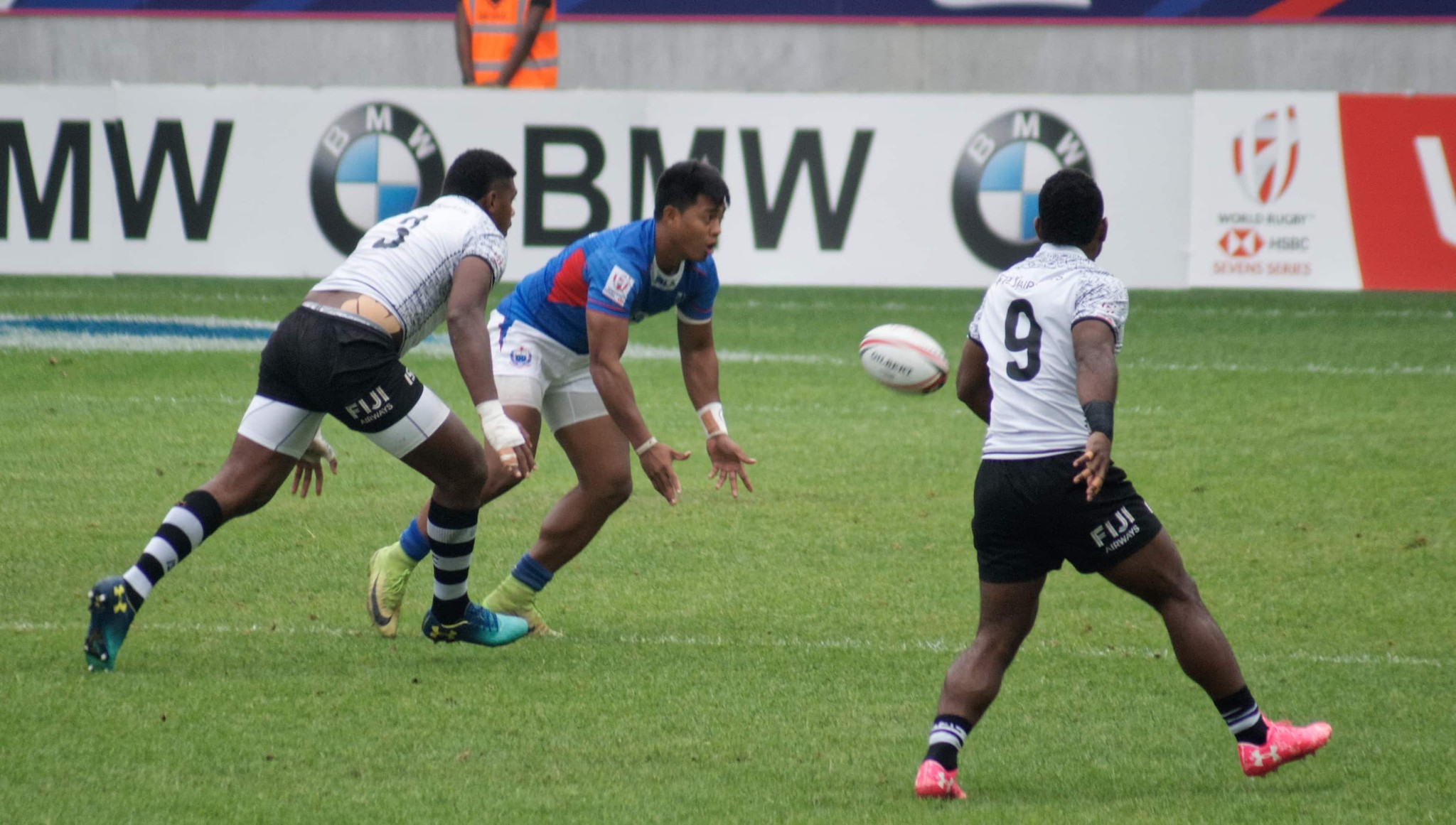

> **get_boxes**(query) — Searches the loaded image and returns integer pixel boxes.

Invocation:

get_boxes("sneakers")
[1237,714,1332,779]
[913,759,966,799]
[83,577,132,672]
[367,546,413,638]
[421,600,529,646]
[481,589,561,636]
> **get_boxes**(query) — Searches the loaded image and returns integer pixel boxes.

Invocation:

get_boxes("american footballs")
[857,323,950,396]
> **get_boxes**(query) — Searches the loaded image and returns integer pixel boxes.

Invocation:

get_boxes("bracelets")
[462,75,474,85]
[634,436,658,457]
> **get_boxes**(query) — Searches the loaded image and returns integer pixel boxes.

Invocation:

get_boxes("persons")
[455,0,561,90]
[85,149,539,673]
[914,168,1332,798]
[366,160,756,640]
